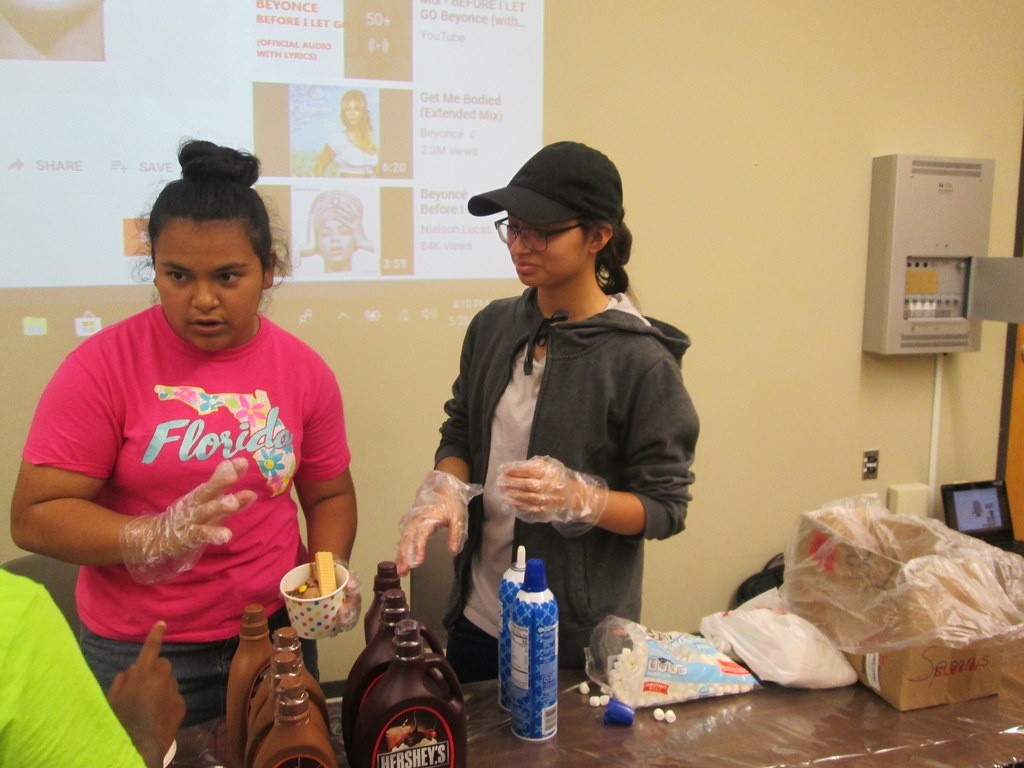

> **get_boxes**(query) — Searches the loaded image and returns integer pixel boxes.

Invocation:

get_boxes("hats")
[467,141,623,223]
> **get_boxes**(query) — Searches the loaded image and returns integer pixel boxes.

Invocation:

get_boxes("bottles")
[508,559,558,742]
[497,545,526,712]
[226,558,468,768]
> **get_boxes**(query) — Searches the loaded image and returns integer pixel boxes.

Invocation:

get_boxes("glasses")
[494,217,584,251]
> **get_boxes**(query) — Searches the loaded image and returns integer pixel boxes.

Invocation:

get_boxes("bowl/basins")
[278,563,349,640]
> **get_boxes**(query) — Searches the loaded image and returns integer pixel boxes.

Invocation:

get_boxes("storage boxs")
[793,513,1002,712]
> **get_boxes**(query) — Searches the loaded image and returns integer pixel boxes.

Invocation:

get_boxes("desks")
[173,635,1024,768]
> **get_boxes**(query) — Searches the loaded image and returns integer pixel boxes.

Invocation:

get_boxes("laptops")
[941,479,1024,557]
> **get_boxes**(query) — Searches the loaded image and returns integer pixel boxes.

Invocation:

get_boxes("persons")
[0,567,186,768]
[9,141,358,729]
[396,142,701,683]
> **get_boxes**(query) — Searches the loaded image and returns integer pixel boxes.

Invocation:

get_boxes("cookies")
[315,551,337,598]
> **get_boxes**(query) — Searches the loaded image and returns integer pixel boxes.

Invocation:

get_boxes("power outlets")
[863,449,879,481]
